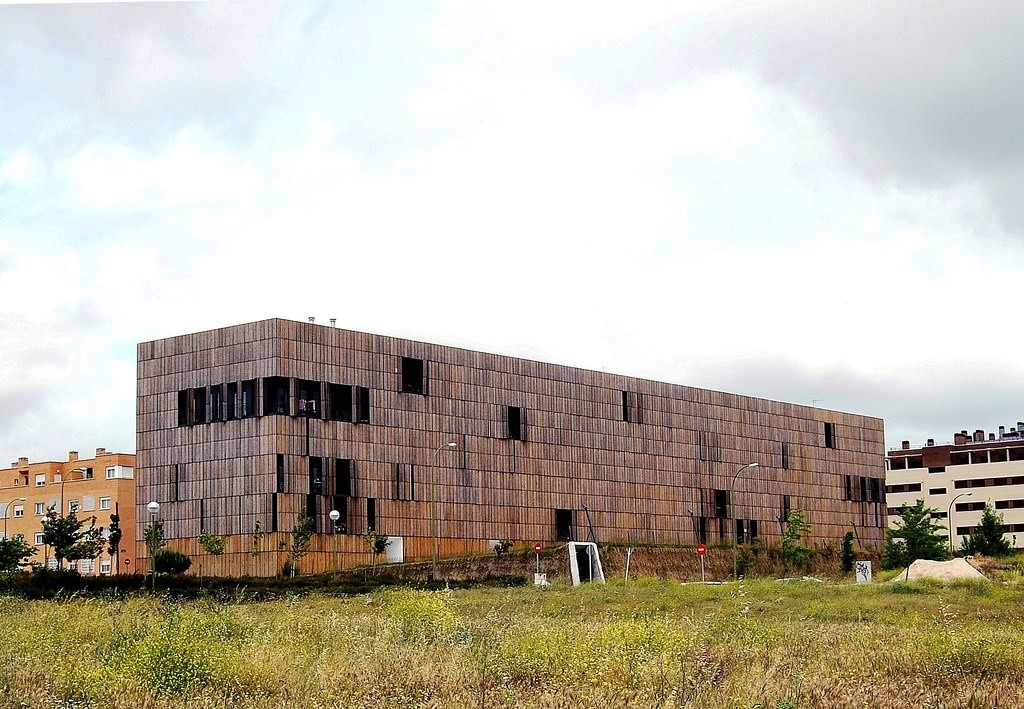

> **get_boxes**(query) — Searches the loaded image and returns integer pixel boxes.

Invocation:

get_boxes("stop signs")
[124,558,131,565]
[534,543,542,554]
[696,544,706,555]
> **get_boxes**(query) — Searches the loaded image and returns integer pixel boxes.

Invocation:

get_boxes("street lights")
[4,497,27,573]
[431,442,458,592]
[948,492,973,559]
[329,510,341,580]
[60,467,88,570]
[731,462,760,579]
[146,500,160,589]
